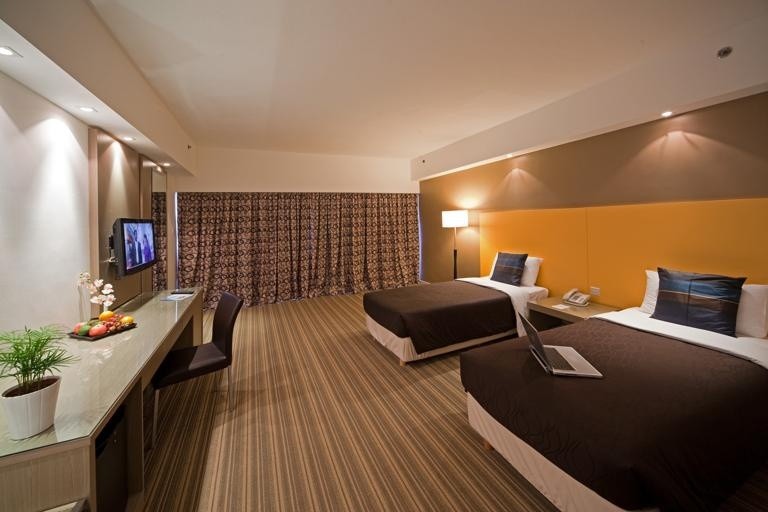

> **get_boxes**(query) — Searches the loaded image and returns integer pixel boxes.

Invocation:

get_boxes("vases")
[104,305,108,311]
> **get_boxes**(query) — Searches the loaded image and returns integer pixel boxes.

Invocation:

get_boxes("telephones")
[561,288,591,308]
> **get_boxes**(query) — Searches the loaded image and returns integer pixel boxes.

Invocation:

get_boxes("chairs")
[148,291,244,447]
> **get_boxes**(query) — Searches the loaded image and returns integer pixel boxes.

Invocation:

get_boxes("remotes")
[171,290,195,295]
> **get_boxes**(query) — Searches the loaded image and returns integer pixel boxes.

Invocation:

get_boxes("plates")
[68,322,138,341]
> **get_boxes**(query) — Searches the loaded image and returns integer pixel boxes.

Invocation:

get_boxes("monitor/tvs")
[108,216,160,276]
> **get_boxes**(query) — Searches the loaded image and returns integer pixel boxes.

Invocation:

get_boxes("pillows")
[489,253,544,287]
[649,267,747,338]
[638,269,768,337]
[490,252,529,286]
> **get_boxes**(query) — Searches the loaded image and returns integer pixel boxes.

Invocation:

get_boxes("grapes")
[101,314,124,332]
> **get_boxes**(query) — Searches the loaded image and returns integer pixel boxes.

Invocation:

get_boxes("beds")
[363,276,549,367]
[459,306,768,512]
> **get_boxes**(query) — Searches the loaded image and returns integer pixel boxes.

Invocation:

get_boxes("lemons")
[120,316,134,324]
[98,310,113,320]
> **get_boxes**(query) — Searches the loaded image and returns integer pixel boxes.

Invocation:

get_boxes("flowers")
[77,272,116,307]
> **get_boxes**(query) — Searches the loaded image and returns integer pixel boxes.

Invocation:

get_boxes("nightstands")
[526,297,624,335]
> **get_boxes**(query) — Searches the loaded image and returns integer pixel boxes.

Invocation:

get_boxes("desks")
[1,286,204,511]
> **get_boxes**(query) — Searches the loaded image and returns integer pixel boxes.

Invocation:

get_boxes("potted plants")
[1,324,82,442]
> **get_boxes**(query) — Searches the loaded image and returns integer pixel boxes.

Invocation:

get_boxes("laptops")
[516,311,602,380]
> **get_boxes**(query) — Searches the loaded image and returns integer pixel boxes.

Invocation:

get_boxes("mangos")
[89,324,106,336]
[78,325,90,335]
[74,322,87,335]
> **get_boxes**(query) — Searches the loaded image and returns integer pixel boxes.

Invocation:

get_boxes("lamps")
[441,210,468,279]
